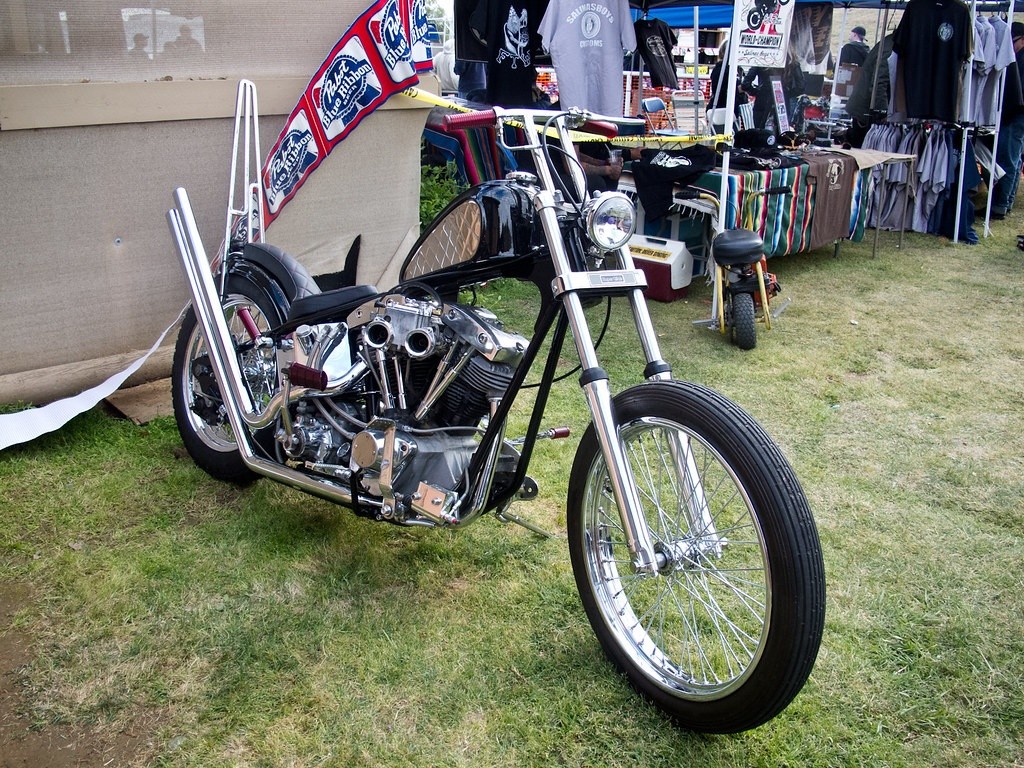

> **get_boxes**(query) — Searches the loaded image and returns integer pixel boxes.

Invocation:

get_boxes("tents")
[454,0,1023,325]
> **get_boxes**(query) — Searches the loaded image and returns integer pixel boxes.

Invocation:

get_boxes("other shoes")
[978,207,1011,220]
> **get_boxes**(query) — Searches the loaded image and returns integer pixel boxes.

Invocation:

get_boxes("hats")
[851,27,868,42]
[133,33,149,43]
[1011,22,1024,43]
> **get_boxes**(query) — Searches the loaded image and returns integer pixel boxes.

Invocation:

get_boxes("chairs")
[706,106,741,138]
[672,90,708,140]
[802,102,854,146]
[642,96,689,150]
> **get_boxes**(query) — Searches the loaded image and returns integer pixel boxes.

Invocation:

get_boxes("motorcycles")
[165,78,826,733]
[675,185,793,348]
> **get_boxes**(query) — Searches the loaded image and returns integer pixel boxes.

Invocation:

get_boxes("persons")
[976,22,1024,220]
[833,26,870,72]
[431,39,460,99]
[706,37,754,135]
[741,40,806,129]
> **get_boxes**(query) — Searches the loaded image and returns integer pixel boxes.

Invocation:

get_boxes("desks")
[812,144,917,258]
[617,152,862,279]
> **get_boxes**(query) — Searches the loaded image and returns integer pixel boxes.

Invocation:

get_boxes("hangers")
[872,109,949,133]
[966,0,1007,17]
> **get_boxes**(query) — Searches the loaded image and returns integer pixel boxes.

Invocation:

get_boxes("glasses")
[1013,37,1023,43]
